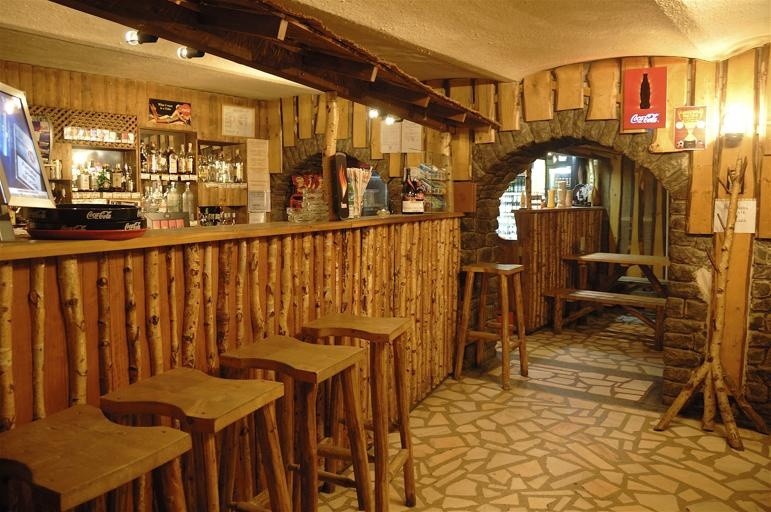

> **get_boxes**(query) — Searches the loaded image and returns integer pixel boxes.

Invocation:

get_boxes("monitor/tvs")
[1,82,56,242]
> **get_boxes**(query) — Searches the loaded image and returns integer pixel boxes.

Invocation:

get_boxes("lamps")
[174,44,205,62]
[121,25,159,48]
[719,102,748,147]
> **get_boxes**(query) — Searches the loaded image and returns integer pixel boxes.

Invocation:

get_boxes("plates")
[25,202,147,239]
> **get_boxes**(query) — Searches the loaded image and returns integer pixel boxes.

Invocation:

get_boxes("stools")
[96,361,292,511]
[298,310,418,512]
[217,330,372,512]
[0,398,194,511]
[451,259,530,392]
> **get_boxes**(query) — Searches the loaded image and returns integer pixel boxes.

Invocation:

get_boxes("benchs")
[541,286,667,353]
[612,276,670,299]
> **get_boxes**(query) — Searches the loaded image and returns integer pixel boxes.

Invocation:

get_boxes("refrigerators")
[544,156,577,206]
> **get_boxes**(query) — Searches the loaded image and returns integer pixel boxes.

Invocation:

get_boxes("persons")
[149,104,188,127]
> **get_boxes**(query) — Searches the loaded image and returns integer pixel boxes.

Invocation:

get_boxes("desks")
[559,251,669,326]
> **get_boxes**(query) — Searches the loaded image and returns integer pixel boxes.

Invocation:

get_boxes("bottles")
[139,140,194,174]
[639,71,652,110]
[552,174,573,186]
[416,182,424,200]
[146,180,194,220]
[404,167,415,200]
[71,160,133,191]
[200,148,244,184]
[499,176,525,239]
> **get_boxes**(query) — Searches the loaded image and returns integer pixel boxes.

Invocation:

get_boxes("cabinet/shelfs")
[416,165,448,213]
[51,122,248,233]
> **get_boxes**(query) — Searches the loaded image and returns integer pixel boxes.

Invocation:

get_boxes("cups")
[547,190,556,207]
[557,182,574,207]
